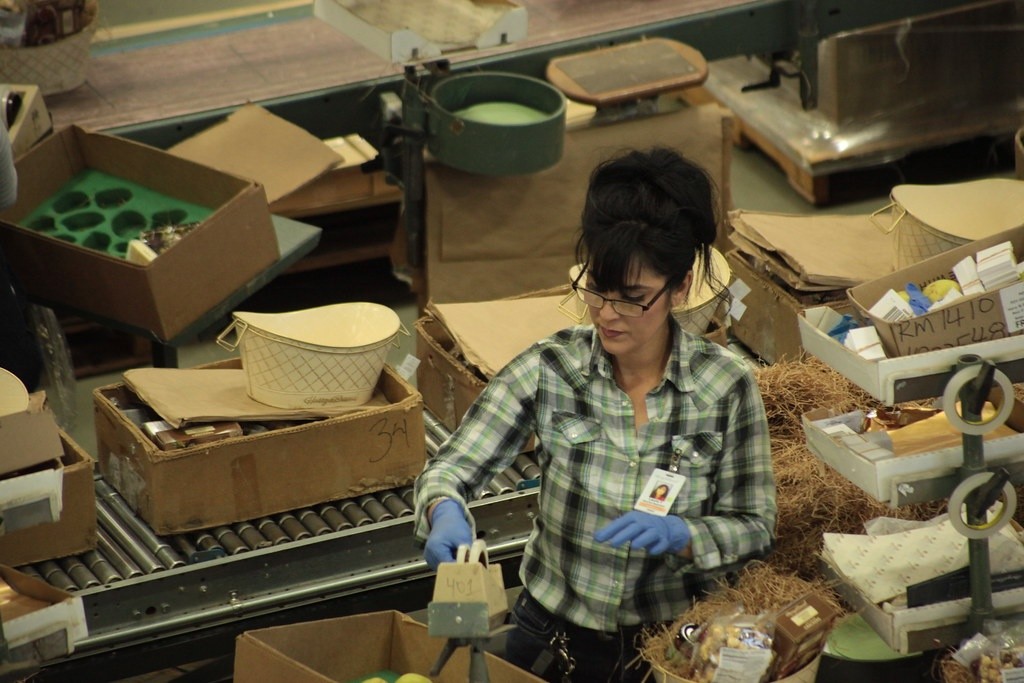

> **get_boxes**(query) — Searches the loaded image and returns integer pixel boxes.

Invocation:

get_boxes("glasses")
[571,251,690,318]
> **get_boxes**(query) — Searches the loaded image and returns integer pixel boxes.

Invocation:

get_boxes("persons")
[412,145,778,683]
[650,484,670,502]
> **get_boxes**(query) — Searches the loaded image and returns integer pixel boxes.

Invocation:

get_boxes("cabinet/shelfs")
[796,299,1024,654]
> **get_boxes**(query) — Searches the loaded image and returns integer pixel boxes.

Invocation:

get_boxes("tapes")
[943,364,1015,435]
[945,470,1017,539]
[455,539,489,568]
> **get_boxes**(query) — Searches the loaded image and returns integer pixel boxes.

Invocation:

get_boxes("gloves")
[422,499,474,570]
[592,508,691,559]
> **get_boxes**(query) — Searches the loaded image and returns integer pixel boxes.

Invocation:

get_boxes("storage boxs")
[234,608,548,682]
[0,122,280,341]
[846,223,1024,357]
[92,357,428,534]
[0,368,97,679]
[712,250,857,366]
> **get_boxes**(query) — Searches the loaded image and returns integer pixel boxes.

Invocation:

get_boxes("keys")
[530,624,576,677]
